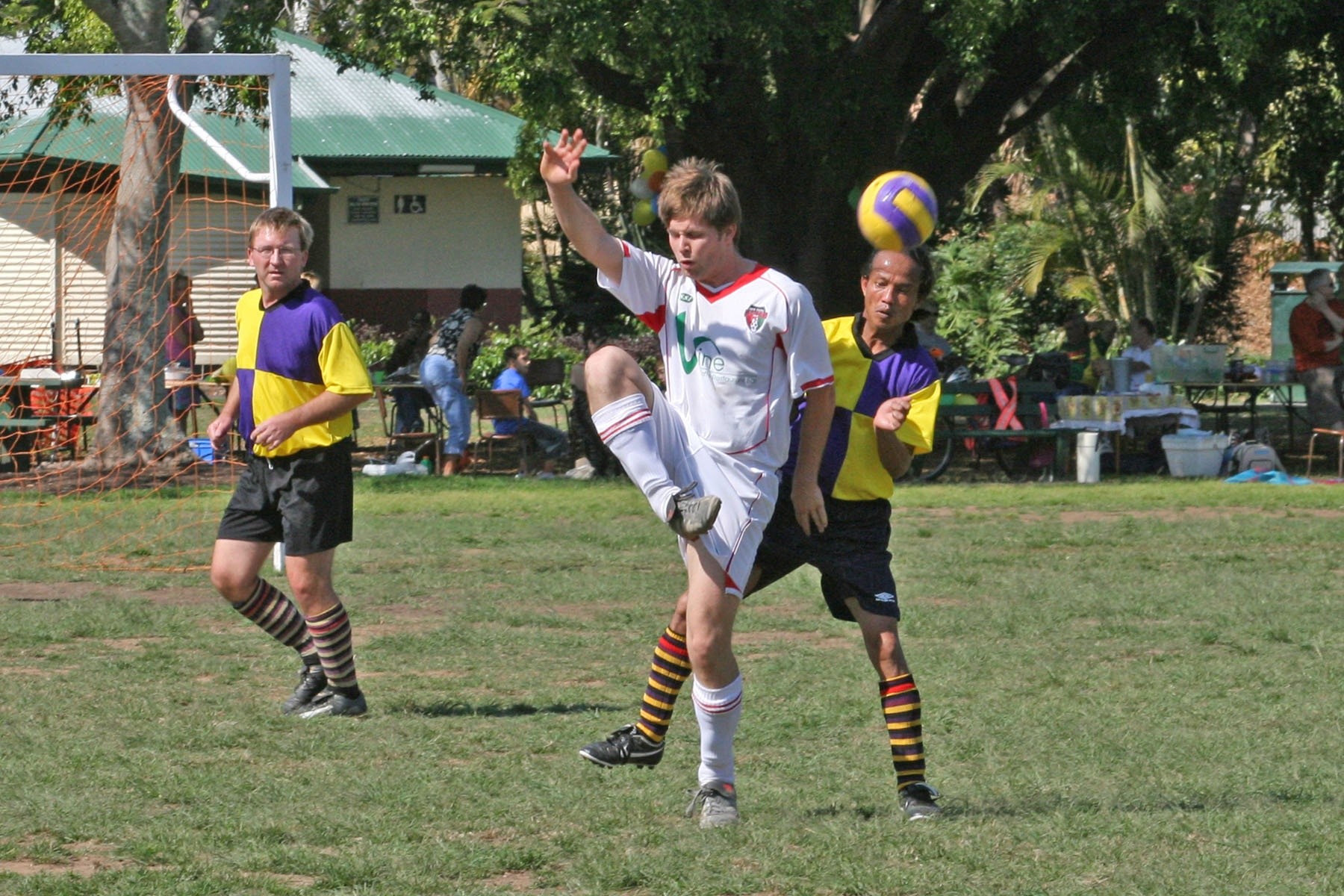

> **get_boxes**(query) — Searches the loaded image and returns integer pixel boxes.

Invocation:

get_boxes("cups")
[374,370,384,384]
[1231,358,1243,383]
[1110,356,1133,393]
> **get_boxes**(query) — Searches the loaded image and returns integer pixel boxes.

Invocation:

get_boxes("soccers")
[857,170,938,252]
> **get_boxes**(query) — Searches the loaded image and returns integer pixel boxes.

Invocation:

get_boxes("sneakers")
[686,780,738,830]
[281,666,327,717]
[296,687,368,722]
[580,724,666,770]
[897,783,944,823]
[666,481,722,542]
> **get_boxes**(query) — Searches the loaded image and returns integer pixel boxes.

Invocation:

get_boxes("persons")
[165,274,205,430]
[1289,263,1344,473]
[386,308,435,450]
[578,245,943,824]
[419,284,491,478]
[539,128,833,831]
[211,205,375,716]
[571,326,667,477]
[491,344,570,480]
[914,299,1175,454]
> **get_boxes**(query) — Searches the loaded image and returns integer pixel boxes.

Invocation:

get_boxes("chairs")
[1293,427,1344,477]
[472,386,541,476]
[385,356,440,431]
[377,387,443,477]
[526,355,574,431]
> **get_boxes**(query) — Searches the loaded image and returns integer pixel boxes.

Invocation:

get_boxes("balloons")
[630,144,667,226]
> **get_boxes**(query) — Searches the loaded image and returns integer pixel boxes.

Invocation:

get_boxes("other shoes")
[443,457,456,478]
[544,461,560,474]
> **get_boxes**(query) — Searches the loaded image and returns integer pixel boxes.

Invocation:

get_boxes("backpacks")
[1225,439,1283,476]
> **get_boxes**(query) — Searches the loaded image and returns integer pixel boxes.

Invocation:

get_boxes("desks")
[0,375,84,476]
[1158,379,1309,464]
[1054,394,1205,477]
[80,379,477,458]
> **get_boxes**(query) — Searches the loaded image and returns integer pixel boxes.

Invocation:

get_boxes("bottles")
[420,456,433,475]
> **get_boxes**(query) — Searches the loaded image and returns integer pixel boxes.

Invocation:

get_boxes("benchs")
[910,381,1083,477]
[0,416,99,465]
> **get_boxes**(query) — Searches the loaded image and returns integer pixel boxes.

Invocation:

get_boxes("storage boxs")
[1150,344,1228,382]
[1162,435,1229,479]
[188,438,218,465]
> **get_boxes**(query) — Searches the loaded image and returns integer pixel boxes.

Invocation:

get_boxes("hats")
[409,311,430,328]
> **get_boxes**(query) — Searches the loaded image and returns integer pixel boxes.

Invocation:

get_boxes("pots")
[163,362,191,380]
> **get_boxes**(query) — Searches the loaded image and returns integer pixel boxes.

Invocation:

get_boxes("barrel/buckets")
[1076,425,1102,483]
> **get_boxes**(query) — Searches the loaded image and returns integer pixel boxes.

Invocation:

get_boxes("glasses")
[249,247,304,258]
[1321,283,1333,289]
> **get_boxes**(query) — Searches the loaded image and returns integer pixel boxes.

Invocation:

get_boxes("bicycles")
[902,333,1057,484]
